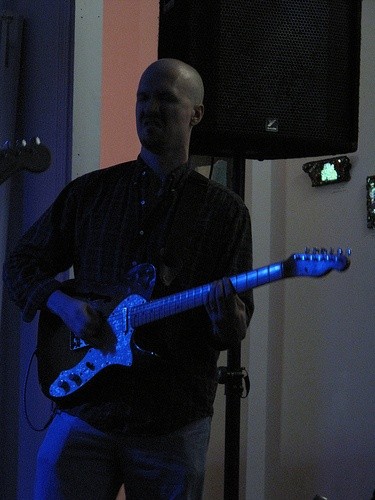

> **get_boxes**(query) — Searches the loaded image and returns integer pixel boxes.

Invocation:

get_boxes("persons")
[4,58,255,499]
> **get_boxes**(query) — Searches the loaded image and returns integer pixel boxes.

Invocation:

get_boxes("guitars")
[35,244,352,404]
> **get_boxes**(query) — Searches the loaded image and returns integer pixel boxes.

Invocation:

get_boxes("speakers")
[159,0,362,162]
